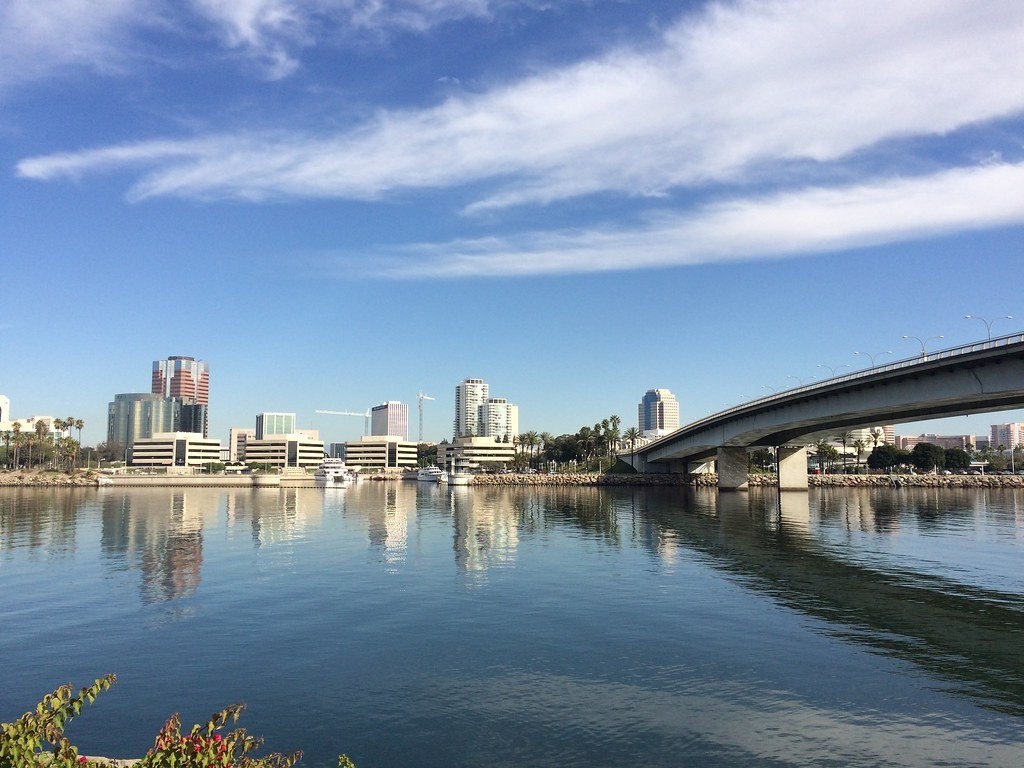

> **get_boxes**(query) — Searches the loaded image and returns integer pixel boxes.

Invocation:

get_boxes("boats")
[416,466,448,482]
[315,457,348,482]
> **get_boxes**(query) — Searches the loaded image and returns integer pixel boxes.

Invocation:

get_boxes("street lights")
[964,314,1013,348]
[901,335,944,355]
[739,395,767,404]
[817,364,850,383]
[786,375,815,391]
[760,385,789,400]
[855,351,893,374]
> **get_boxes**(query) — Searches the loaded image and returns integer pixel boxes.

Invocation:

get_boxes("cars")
[937,468,1024,475]
